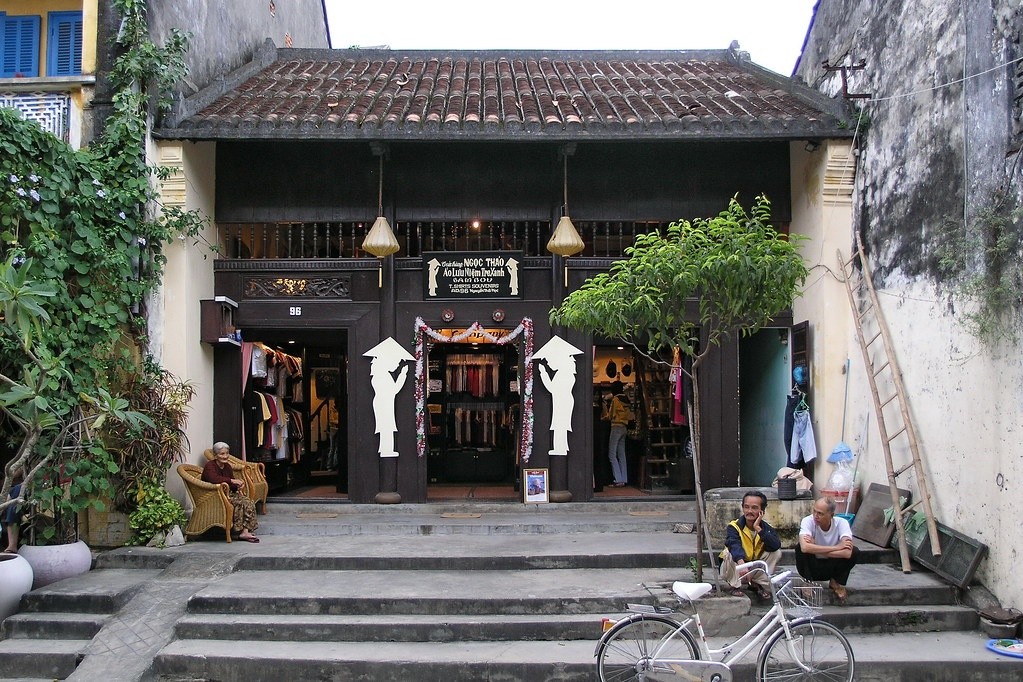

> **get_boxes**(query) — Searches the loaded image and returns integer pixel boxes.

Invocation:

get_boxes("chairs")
[177,463,249,543]
[204,448,268,514]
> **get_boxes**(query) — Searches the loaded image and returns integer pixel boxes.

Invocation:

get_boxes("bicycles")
[594,561,857,682]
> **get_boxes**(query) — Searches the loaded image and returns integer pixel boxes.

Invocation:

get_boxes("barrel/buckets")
[819,486,860,514]
[834,513,855,528]
[778,477,796,499]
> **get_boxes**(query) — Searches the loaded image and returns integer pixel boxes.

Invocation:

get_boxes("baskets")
[778,576,824,617]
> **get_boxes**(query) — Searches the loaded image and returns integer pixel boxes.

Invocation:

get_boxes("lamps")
[546,153,585,287]
[361,144,399,288]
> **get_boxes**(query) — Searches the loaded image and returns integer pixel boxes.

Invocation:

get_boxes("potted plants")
[0,250,156,629]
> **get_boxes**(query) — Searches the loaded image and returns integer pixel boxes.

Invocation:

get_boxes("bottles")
[235,329,242,341]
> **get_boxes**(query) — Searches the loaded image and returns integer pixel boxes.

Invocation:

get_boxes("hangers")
[791,383,801,395]
[795,393,810,411]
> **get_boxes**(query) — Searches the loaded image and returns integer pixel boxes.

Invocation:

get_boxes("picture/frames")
[523,468,549,504]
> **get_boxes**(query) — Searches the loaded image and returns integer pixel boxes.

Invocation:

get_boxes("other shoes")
[608,482,624,487]
[729,586,743,596]
[829,582,847,600]
[801,583,813,598]
[747,584,771,600]
[238,534,260,543]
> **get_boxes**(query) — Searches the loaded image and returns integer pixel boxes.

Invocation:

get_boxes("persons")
[794,495,859,600]
[3,484,31,554]
[199,441,259,544]
[718,490,781,599]
[600,381,629,487]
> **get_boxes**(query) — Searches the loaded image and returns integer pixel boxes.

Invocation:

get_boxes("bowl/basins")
[981,616,1020,640]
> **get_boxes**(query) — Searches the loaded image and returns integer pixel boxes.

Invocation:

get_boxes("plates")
[986,638,1023,658]
